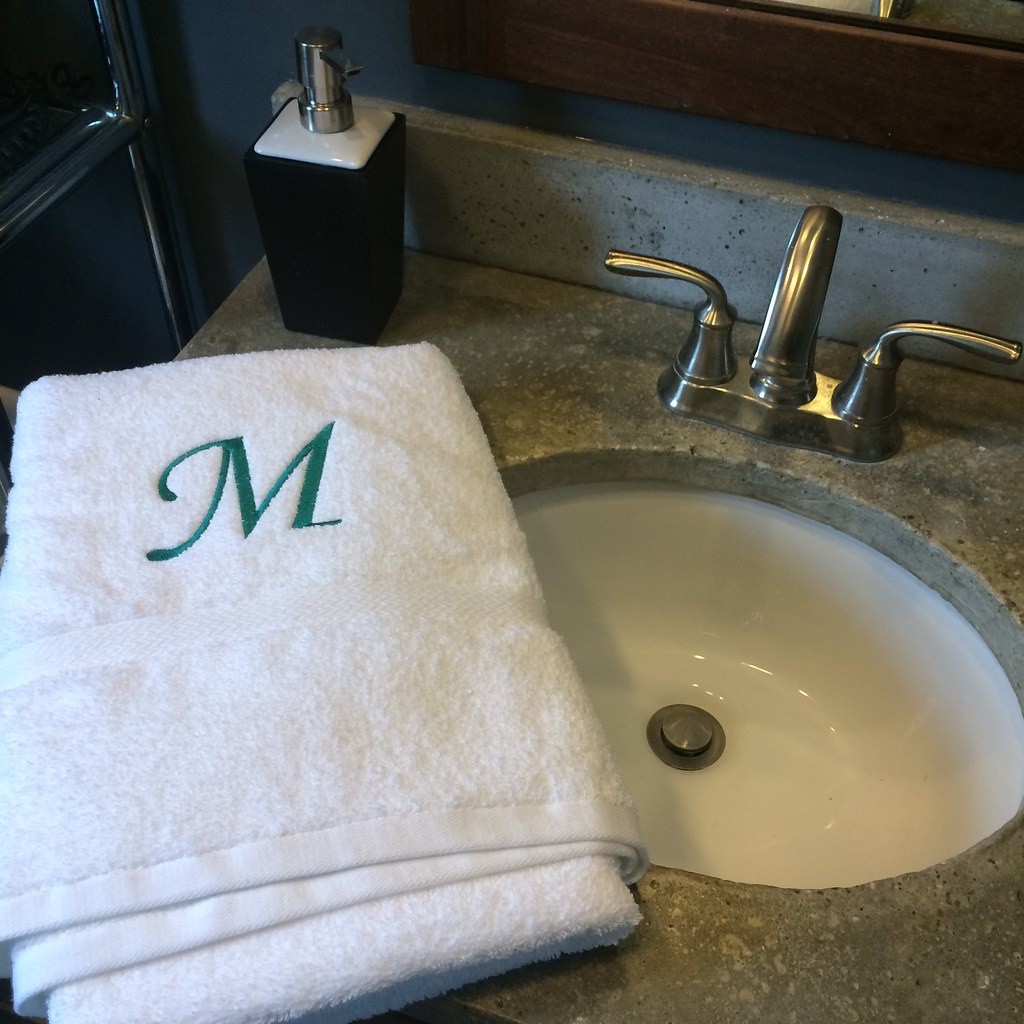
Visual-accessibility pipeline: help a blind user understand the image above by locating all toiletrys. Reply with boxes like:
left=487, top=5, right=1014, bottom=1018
left=244, top=27, right=405, bottom=343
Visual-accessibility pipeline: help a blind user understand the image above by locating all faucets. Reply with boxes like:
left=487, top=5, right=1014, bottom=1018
left=748, top=203, right=843, bottom=410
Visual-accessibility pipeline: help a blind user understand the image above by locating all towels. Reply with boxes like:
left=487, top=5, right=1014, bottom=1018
left=1, top=344, right=653, bottom=1016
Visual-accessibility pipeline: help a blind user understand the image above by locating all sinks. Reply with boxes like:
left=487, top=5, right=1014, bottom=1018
left=499, top=447, right=1024, bottom=892
